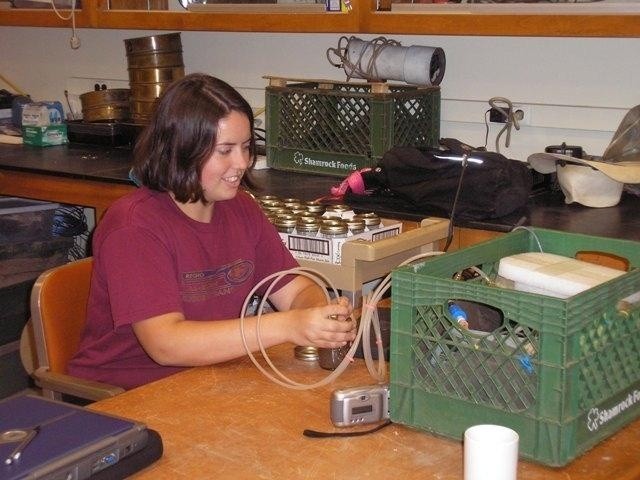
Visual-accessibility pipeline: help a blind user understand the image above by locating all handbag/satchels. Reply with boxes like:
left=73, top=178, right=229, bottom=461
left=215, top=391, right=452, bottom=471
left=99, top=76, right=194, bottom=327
left=345, top=146, right=527, bottom=223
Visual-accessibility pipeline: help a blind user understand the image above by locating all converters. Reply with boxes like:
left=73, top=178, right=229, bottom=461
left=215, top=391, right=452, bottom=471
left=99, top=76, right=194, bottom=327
left=490, top=107, right=510, bottom=123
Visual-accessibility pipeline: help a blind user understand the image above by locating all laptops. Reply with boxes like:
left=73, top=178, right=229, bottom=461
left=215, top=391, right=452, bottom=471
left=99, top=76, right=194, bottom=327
left=0, top=392, right=149, bottom=480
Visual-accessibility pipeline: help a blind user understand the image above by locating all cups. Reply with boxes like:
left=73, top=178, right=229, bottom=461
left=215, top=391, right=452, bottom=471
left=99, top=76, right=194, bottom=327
left=463, top=424, right=519, bottom=480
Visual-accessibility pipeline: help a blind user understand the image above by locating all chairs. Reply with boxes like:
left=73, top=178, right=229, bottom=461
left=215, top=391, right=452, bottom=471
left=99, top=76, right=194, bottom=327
left=17, top=257, right=131, bottom=408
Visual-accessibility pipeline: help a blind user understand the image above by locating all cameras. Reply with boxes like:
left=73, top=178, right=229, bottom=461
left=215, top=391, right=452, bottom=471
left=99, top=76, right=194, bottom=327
left=331, top=383, right=389, bottom=428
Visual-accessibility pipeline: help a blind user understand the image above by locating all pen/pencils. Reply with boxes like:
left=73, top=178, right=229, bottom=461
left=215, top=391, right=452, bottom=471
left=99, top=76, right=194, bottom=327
left=4, top=425, right=41, bottom=466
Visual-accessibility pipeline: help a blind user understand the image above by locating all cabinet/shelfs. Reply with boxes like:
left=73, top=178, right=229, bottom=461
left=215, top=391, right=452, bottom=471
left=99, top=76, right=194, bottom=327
left=359, top=0, right=640, bottom=38
left=0, top=1, right=94, bottom=31
left=94, top=0, right=359, bottom=36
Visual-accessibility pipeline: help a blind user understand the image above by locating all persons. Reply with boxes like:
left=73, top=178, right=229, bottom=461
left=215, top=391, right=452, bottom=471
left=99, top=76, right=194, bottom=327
left=62, top=73, right=357, bottom=407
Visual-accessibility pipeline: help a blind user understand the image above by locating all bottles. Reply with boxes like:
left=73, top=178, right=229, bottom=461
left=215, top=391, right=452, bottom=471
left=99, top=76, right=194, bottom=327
left=317, top=313, right=350, bottom=371
left=257, top=194, right=382, bottom=239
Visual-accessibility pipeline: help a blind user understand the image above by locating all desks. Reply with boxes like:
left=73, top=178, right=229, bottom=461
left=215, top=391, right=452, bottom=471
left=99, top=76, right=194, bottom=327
left=77, top=294, right=639, bottom=480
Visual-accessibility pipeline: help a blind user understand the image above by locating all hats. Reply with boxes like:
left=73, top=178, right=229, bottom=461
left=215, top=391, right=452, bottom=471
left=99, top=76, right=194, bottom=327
left=529, top=153, right=640, bottom=207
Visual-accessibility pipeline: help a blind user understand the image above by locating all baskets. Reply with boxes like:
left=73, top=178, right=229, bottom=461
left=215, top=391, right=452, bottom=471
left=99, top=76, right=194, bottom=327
left=264, top=81, right=440, bottom=177
left=389, top=226, right=639, bottom=469
